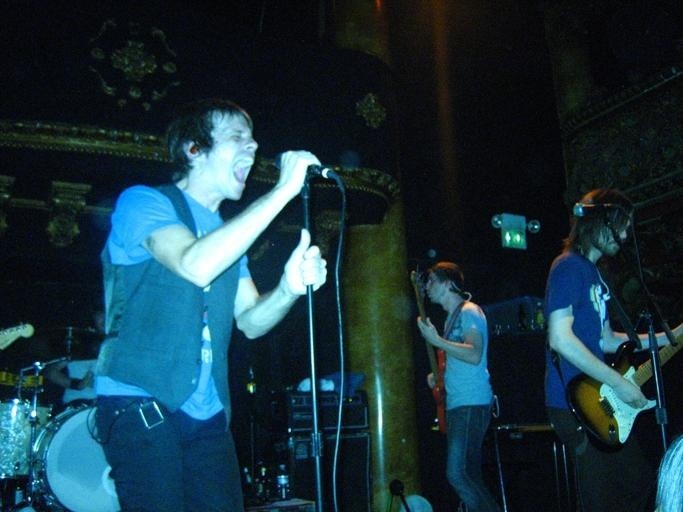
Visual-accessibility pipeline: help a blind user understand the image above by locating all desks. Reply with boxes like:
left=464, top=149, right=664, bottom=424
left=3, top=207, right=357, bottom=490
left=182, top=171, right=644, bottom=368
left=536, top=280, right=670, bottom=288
left=491, top=420, right=581, bottom=512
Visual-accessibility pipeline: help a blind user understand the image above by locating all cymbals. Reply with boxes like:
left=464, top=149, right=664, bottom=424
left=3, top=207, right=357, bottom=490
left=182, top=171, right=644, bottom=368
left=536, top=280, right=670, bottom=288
left=48, top=322, right=92, bottom=340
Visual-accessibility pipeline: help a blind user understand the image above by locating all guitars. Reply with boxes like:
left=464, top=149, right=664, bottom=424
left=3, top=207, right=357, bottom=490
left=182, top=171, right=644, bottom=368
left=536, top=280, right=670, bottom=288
left=410, top=272, right=446, bottom=433
left=565, top=333, right=682, bottom=452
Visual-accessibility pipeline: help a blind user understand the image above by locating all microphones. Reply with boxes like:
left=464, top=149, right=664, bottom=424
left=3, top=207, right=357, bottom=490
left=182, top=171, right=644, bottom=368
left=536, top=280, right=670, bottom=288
left=274, top=153, right=335, bottom=181
left=573, top=203, right=616, bottom=220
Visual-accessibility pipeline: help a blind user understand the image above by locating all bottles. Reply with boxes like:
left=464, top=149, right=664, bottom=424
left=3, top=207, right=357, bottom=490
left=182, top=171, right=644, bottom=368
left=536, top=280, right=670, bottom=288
left=13, top=463, right=26, bottom=505
left=535, top=302, right=546, bottom=330
left=241, top=465, right=289, bottom=502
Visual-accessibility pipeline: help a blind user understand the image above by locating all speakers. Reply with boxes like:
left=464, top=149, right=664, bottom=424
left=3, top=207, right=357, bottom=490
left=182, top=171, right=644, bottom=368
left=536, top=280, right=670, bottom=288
left=258, top=388, right=373, bottom=511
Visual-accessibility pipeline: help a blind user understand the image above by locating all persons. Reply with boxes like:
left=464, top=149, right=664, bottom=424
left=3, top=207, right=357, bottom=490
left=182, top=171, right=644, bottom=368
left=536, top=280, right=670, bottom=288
left=544, top=188, right=658, bottom=512
left=87, top=99, right=329, bottom=512
left=228, top=328, right=256, bottom=503
left=416, top=262, right=502, bottom=511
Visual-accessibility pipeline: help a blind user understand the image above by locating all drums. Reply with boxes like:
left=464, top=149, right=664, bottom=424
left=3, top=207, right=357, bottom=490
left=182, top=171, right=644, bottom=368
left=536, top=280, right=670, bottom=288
left=1, top=398, right=54, bottom=479
left=30, top=406, right=123, bottom=512
